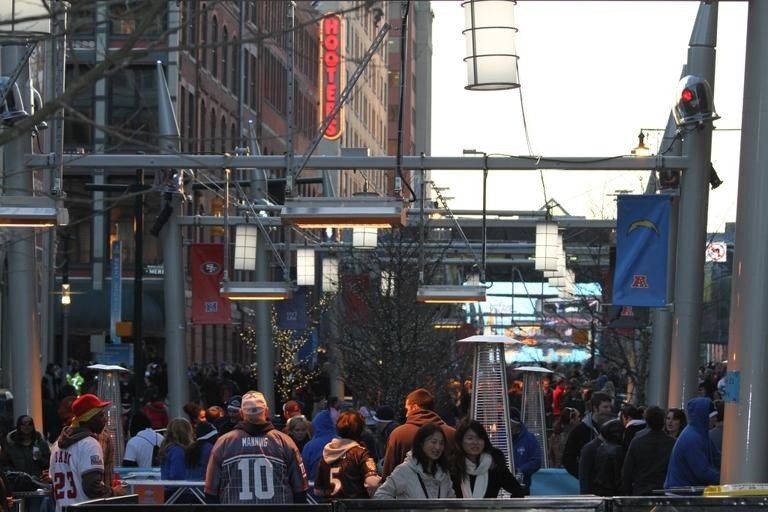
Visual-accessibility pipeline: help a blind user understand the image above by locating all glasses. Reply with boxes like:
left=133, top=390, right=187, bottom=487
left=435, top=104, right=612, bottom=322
left=20, top=421, right=33, bottom=427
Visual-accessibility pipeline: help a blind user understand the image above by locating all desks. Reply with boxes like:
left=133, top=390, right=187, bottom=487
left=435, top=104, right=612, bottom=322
left=119, top=479, right=208, bottom=505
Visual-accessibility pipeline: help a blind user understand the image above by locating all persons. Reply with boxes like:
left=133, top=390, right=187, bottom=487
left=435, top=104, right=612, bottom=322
left=0, top=358, right=127, bottom=512
left=123, top=370, right=525, bottom=512
left=507, top=368, right=729, bottom=497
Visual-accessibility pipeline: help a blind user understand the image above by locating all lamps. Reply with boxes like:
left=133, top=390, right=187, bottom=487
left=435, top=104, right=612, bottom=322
left=417, top=153, right=487, bottom=303
left=234, top=225, right=257, bottom=271
left=381, top=271, right=395, bottom=297
left=297, top=239, right=315, bottom=285
left=280, top=0, right=403, bottom=228
left=323, top=258, right=339, bottom=292
left=535, top=209, right=575, bottom=299
left=433, top=320, right=464, bottom=328
left=353, top=228, right=377, bottom=249
left=0, top=0, right=69, bottom=227
left=460, top=0, right=521, bottom=91
left=223, top=168, right=292, bottom=301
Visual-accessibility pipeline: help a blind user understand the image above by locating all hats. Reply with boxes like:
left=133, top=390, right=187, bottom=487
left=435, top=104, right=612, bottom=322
left=227, top=394, right=242, bottom=410
left=71, top=393, right=113, bottom=418
left=241, top=390, right=268, bottom=414
left=709, top=401, right=719, bottom=419
left=195, top=420, right=219, bottom=441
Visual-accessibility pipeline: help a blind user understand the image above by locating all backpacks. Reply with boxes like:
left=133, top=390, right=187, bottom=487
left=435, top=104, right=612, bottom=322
left=136, top=432, right=160, bottom=467
